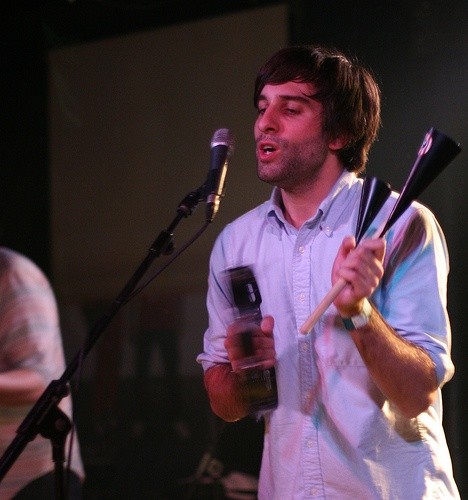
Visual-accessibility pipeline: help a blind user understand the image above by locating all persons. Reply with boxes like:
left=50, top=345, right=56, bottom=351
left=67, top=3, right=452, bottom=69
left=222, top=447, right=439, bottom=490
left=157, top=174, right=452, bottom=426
left=195, top=44, right=462, bottom=500
left=0, top=243, right=86, bottom=500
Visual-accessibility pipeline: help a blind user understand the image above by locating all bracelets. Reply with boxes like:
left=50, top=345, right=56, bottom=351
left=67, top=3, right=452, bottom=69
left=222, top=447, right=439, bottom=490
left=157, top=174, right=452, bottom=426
left=339, top=296, right=374, bottom=332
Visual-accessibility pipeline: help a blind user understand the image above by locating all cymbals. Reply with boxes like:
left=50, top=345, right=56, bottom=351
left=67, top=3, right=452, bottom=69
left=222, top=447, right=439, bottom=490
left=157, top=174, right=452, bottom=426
left=223, top=265, right=278, bottom=410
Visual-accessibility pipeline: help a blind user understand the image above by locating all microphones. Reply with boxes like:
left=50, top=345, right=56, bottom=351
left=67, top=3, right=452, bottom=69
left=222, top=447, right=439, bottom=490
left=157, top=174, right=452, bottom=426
left=228, top=267, right=277, bottom=413
left=206, top=128, right=235, bottom=221
